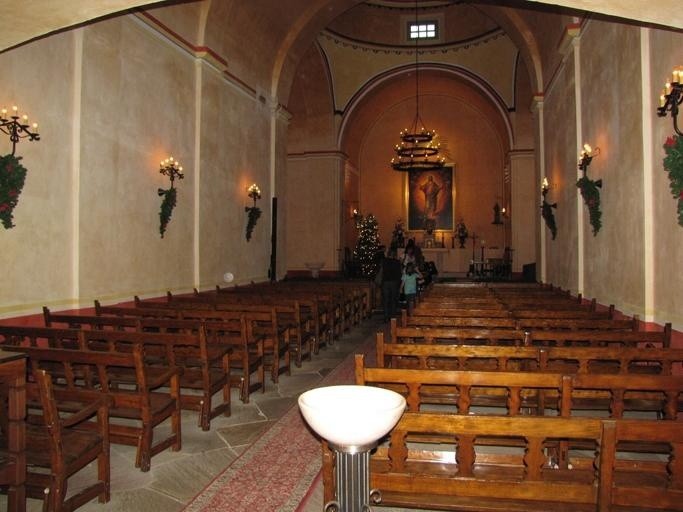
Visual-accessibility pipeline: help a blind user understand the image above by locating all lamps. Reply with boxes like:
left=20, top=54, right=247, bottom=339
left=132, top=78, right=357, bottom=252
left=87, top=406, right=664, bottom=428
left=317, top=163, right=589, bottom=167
left=574, top=142, right=603, bottom=189
left=538, top=177, right=558, bottom=209
left=0, top=106, right=41, bottom=159
left=153, top=156, right=185, bottom=196
left=244, top=183, right=263, bottom=212
left=657, top=64, right=682, bottom=136
left=389, top=1, right=445, bottom=172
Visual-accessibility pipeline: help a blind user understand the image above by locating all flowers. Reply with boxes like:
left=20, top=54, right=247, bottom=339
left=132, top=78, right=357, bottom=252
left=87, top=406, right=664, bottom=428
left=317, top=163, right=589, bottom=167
left=156, top=187, right=178, bottom=239
left=0, top=155, right=27, bottom=229
left=662, top=134, right=682, bottom=227
left=244, top=207, right=262, bottom=243
left=541, top=200, right=557, bottom=240
left=576, top=175, right=602, bottom=237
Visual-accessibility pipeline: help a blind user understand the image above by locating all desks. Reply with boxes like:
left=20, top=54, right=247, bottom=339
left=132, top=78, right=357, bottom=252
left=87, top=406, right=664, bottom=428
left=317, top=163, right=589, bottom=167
left=396, top=246, right=473, bottom=274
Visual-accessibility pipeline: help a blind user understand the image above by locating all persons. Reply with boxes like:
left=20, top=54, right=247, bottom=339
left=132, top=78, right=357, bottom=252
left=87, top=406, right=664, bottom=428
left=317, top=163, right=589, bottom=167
left=416, top=176, right=448, bottom=219
left=454, top=218, right=468, bottom=240
left=392, top=217, right=405, bottom=236
left=373, top=238, right=425, bottom=324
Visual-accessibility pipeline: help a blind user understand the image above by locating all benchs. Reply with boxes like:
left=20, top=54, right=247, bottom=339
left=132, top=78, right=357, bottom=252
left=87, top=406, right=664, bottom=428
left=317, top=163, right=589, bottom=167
left=1, top=300, right=266, bottom=512
left=322, top=278, right=682, bottom=512
left=135, top=276, right=370, bottom=384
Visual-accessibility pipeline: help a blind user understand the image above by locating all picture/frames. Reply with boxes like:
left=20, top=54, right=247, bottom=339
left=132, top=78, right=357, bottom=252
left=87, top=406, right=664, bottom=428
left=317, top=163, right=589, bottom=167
left=401, top=162, right=457, bottom=233
